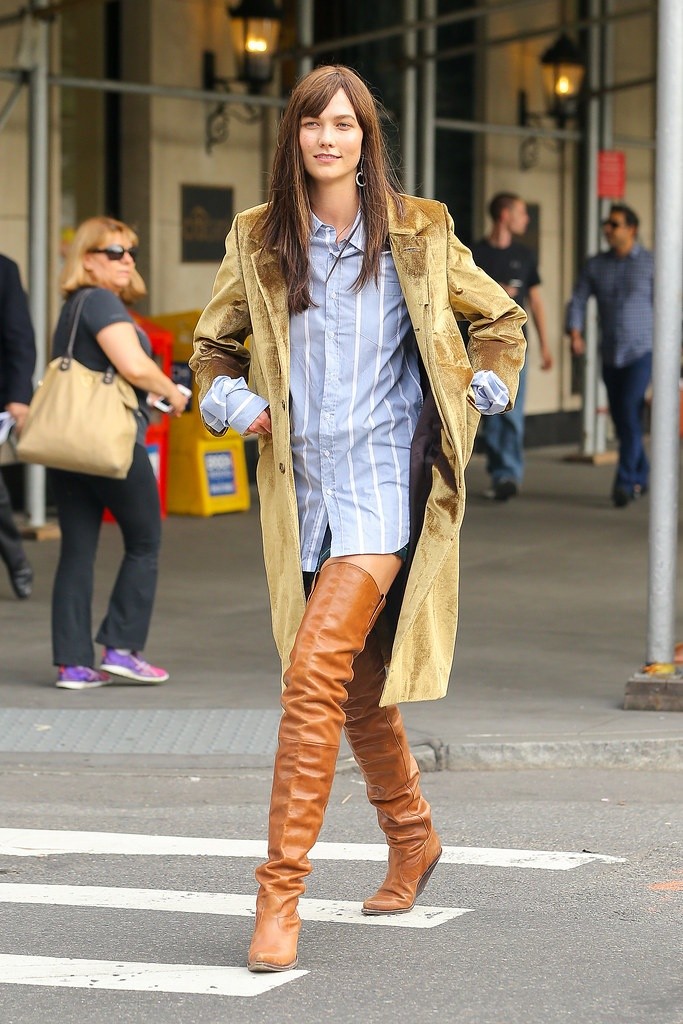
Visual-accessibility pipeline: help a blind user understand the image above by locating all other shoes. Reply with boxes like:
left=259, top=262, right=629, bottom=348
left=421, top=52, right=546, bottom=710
left=613, top=488, right=632, bottom=507
left=8, top=561, right=33, bottom=600
left=493, top=479, right=516, bottom=502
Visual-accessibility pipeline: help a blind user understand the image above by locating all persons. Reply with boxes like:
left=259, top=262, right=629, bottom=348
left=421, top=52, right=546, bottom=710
left=466, top=194, right=551, bottom=502
left=0, top=255, right=36, bottom=599
left=190, top=65, right=527, bottom=971
left=565, top=206, right=651, bottom=505
left=51, top=218, right=188, bottom=689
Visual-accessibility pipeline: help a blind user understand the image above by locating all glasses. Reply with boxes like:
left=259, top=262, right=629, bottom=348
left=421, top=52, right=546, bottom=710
left=603, top=218, right=620, bottom=229
left=87, top=244, right=142, bottom=262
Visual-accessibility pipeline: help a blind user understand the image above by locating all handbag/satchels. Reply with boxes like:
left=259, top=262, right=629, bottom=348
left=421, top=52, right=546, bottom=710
left=14, top=356, right=151, bottom=480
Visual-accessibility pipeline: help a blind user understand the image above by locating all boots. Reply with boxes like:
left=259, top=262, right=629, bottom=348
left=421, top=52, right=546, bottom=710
left=348, top=636, right=443, bottom=917
left=245, top=561, right=386, bottom=972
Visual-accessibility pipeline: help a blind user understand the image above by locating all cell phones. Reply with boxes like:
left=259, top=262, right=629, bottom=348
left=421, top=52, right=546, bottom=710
left=153, top=384, right=192, bottom=413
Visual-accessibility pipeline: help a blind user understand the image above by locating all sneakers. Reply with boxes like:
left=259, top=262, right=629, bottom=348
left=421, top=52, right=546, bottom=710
left=55, top=664, right=113, bottom=690
left=99, top=647, right=170, bottom=682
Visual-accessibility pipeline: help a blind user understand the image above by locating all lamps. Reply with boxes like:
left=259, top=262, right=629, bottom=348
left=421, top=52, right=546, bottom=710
left=517, top=30, right=590, bottom=170
left=198, top=0, right=302, bottom=156
